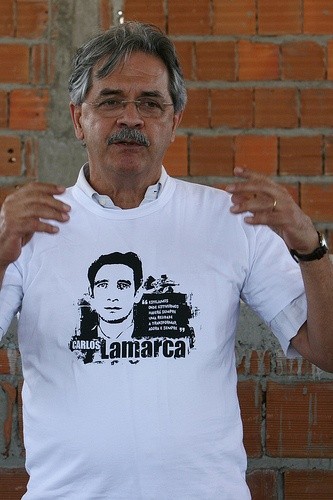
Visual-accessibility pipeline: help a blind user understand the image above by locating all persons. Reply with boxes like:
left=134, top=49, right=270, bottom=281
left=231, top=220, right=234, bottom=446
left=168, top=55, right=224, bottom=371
left=0, top=22, right=333, bottom=500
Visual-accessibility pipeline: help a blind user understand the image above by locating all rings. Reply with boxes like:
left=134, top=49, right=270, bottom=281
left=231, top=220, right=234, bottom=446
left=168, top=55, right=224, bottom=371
left=271, top=200, right=278, bottom=212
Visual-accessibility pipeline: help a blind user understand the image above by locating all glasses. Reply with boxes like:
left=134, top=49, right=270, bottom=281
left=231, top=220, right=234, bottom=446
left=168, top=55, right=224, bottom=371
left=81, top=96, right=175, bottom=119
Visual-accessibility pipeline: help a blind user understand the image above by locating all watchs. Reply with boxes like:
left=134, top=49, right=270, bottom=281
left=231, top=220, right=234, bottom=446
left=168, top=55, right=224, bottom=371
left=289, top=231, right=328, bottom=263
